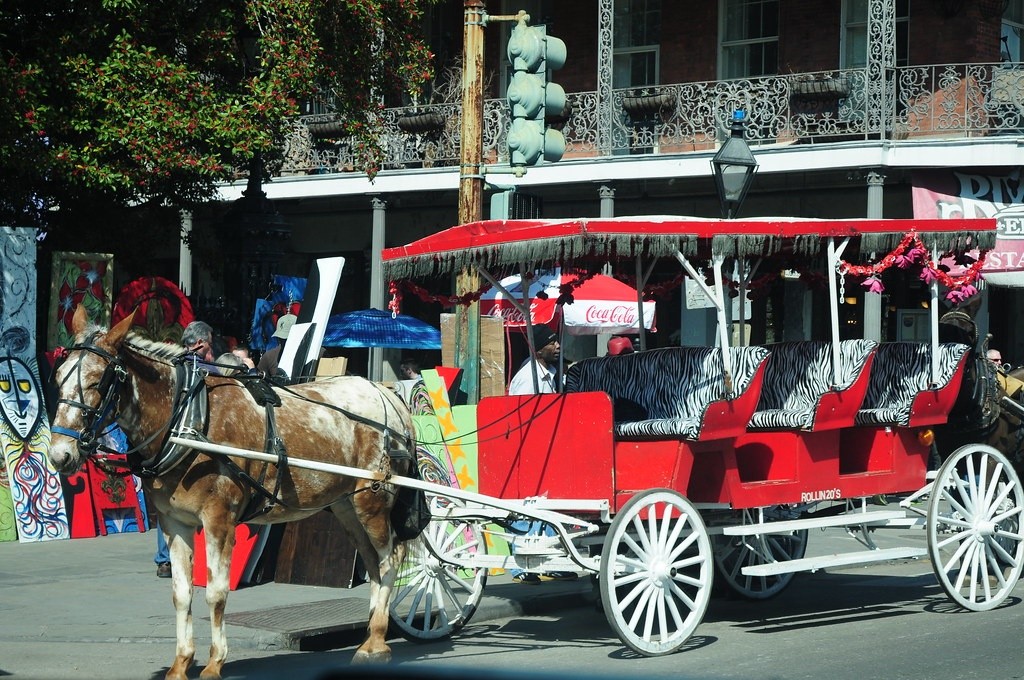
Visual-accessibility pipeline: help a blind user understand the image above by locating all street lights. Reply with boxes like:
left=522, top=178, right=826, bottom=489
left=709, top=109, right=760, bottom=350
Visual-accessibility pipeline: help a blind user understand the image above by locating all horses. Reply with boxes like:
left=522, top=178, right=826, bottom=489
left=47, top=303, right=418, bottom=680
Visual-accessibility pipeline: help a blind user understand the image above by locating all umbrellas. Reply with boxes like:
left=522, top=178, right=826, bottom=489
left=323, top=307, right=442, bottom=381
left=480, top=262, right=656, bottom=393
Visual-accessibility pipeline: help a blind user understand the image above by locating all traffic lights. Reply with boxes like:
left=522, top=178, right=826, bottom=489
left=506, top=23, right=568, bottom=167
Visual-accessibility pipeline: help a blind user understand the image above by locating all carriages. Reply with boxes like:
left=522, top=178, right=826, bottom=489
left=45, top=215, right=1024, bottom=680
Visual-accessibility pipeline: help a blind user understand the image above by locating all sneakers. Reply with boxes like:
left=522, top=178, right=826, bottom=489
left=157, top=562, right=171, bottom=577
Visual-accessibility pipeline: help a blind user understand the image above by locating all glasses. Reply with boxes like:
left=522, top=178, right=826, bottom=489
left=990, top=359, right=1002, bottom=362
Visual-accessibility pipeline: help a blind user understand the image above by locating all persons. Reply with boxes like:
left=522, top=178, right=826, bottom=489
left=400, top=358, right=423, bottom=380
left=986, top=349, right=1002, bottom=367
left=258, top=314, right=297, bottom=375
left=509, top=323, right=560, bottom=396
left=153, top=320, right=220, bottom=577
left=214, top=344, right=256, bottom=377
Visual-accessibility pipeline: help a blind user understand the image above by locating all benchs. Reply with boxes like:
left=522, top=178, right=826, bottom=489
left=562, top=340, right=972, bottom=444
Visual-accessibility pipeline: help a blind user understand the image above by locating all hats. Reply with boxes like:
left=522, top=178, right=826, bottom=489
left=271, top=314, right=297, bottom=339
left=533, top=325, right=558, bottom=352
